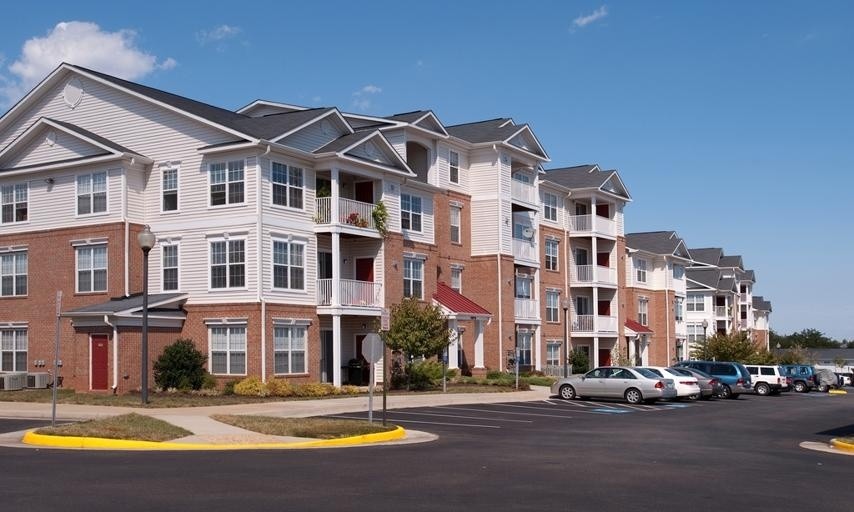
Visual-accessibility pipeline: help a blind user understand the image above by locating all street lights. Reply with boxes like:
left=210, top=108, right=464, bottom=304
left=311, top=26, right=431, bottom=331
left=701, top=319, right=708, bottom=361
left=776, top=342, right=781, bottom=365
left=136, top=224, right=156, bottom=403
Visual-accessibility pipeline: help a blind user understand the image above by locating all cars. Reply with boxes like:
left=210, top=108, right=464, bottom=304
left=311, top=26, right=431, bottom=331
left=550, top=361, right=792, bottom=404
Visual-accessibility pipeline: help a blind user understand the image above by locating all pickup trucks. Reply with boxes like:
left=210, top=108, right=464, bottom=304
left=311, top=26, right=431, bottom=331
left=781, top=365, right=844, bottom=392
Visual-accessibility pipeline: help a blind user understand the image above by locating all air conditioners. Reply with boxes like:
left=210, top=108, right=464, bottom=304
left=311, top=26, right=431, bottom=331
left=0, top=373, right=22, bottom=390
left=26, top=372, right=48, bottom=388
